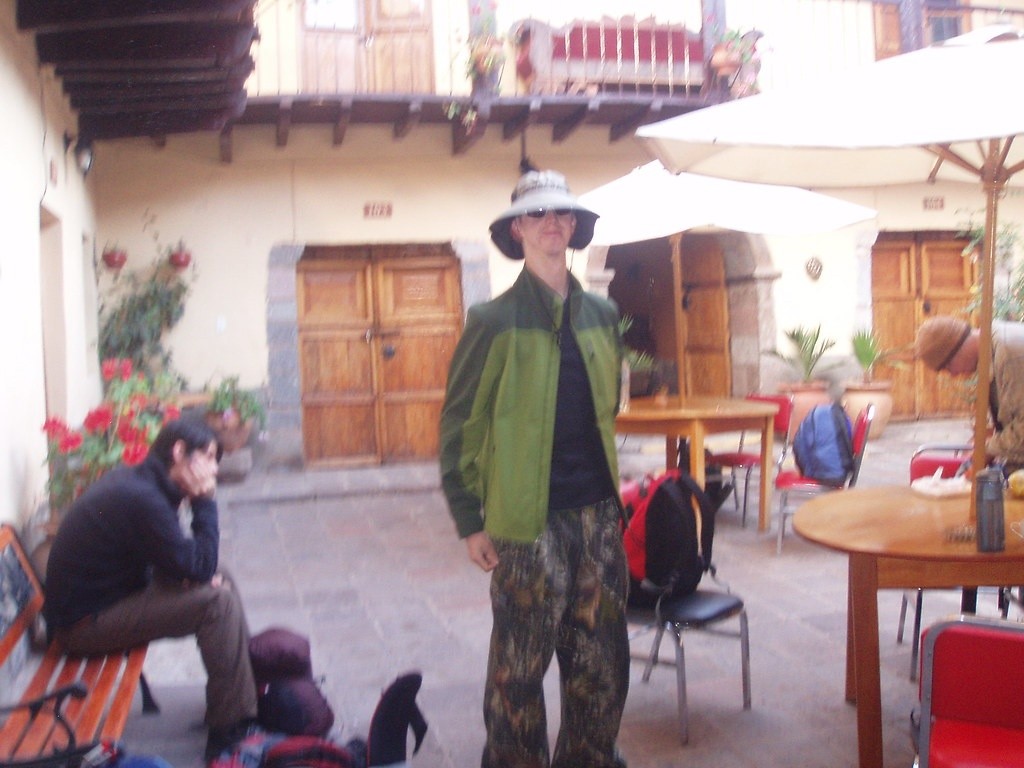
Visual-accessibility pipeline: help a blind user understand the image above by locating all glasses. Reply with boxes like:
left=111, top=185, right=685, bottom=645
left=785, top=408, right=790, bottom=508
left=525, top=205, right=573, bottom=218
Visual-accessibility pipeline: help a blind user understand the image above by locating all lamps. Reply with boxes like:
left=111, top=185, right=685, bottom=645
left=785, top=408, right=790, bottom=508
left=77, top=135, right=96, bottom=177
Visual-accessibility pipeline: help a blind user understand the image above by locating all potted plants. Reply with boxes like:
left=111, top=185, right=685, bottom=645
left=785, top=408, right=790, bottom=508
left=767, top=330, right=834, bottom=444
left=842, top=333, right=907, bottom=439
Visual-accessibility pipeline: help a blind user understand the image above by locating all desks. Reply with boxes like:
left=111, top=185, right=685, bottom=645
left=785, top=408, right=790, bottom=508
left=793, top=488, right=1024, bottom=767
left=607, top=393, right=777, bottom=535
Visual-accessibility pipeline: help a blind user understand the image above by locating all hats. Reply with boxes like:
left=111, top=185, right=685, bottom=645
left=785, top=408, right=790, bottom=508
left=489, top=169, right=599, bottom=261
left=916, top=318, right=973, bottom=373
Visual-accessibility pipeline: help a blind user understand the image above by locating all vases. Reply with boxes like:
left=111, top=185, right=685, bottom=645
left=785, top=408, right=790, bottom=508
left=29, top=515, right=63, bottom=580
left=207, top=408, right=251, bottom=452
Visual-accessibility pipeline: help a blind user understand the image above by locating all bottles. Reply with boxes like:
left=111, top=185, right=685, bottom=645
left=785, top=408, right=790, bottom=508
left=976, top=462, right=1006, bottom=553
left=619, top=357, right=631, bottom=414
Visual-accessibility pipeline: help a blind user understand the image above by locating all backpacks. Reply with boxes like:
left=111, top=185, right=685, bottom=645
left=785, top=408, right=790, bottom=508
left=791, top=401, right=857, bottom=490
left=207, top=671, right=429, bottom=766
left=248, top=628, right=334, bottom=738
left=620, top=467, right=717, bottom=611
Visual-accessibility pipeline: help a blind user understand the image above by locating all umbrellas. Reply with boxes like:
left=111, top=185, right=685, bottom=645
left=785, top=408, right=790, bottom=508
left=573, top=27, right=1024, bottom=482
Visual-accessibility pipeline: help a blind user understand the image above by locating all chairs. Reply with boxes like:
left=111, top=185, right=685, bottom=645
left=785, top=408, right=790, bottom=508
left=704, top=392, right=793, bottom=529
left=896, top=443, right=976, bottom=640
left=910, top=613, right=1024, bottom=768
left=774, top=405, right=877, bottom=548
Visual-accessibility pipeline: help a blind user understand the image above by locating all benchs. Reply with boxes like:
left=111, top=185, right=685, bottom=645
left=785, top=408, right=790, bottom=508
left=507, top=17, right=716, bottom=92
left=0, top=524, right=161, bottom=768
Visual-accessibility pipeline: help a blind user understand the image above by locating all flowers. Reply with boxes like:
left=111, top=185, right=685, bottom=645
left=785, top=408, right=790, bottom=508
left=34, top=250, right=194, bottom=524
left=208, top=376, right=268, bottom=442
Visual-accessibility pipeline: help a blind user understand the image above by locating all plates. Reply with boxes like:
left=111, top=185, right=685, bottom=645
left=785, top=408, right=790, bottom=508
left=911, top=477, right=972, bottom=497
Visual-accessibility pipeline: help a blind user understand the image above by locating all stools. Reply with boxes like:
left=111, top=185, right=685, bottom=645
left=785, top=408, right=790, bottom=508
left=624, top=589, right=750, bottom=751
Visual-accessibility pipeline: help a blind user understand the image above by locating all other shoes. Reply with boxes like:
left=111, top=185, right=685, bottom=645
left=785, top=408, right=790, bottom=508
left=204, top=720, right=257, bottom=761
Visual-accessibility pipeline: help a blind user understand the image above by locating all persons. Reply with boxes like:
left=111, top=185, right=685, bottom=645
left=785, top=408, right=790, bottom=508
left=438, top=171, right=632, bottom=768
left=41, top=419, right=260, bottom=761
left=915, top=315, right=1024, bottom=483
